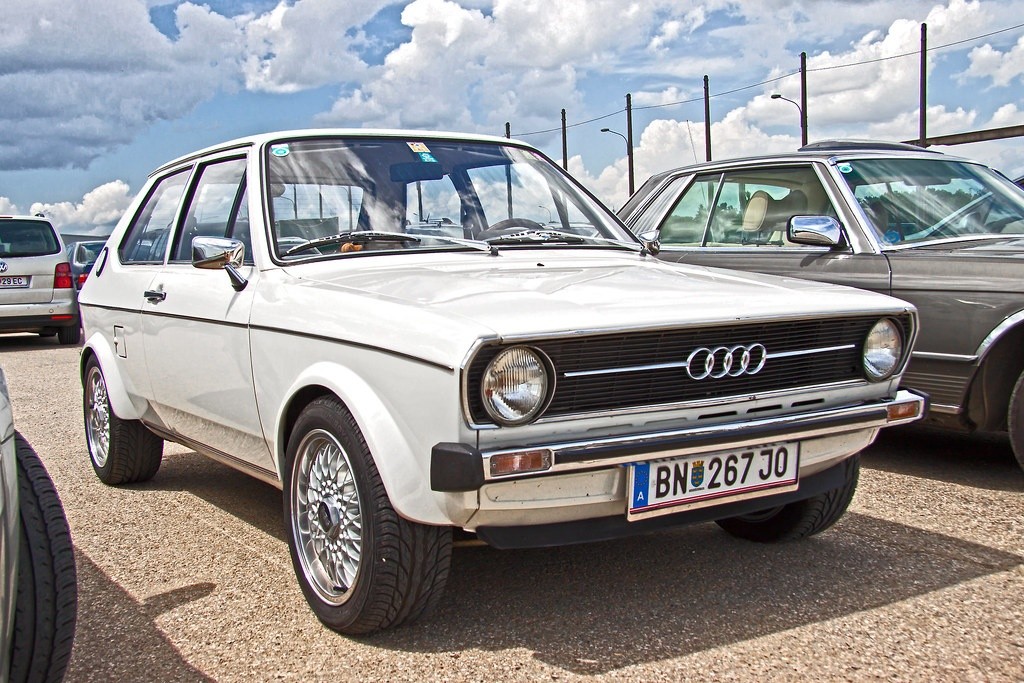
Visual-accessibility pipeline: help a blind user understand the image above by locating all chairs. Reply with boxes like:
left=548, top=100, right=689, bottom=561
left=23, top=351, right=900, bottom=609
left=1, top=214, right=1024, bottom=256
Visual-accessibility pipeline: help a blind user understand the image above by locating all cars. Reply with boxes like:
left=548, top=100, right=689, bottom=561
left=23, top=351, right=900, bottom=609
left=591, top=138, right=1024, bottom=479
left=71, top=128, right=931, bottom=640
left=65, top=239, right=107, bottom=291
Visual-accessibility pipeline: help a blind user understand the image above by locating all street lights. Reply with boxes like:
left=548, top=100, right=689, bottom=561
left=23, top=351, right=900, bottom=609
left=770, top=94, right=810, bottom=147
left=601, top=127, right=639, bottom=196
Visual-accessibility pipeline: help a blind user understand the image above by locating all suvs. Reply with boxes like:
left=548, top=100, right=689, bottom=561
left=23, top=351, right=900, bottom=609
left=0, top=212, right=80, bottom=345
